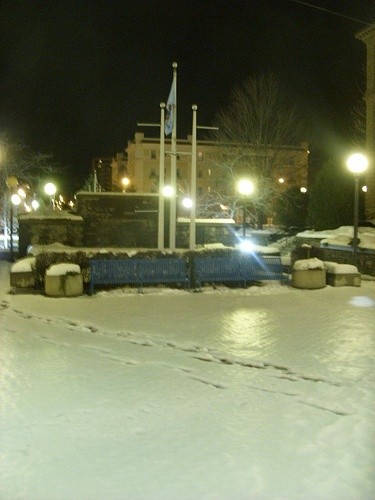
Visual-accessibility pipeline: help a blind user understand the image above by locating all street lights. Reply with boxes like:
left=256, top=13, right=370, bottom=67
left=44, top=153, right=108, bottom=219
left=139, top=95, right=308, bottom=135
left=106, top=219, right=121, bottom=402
left=346, top=152, right=370, bottom=267
left=44, top=183, right=56, bottom=210
left=237, top=180, right=254, bottom=239
left=6, top=176, right=18, bottom=262
